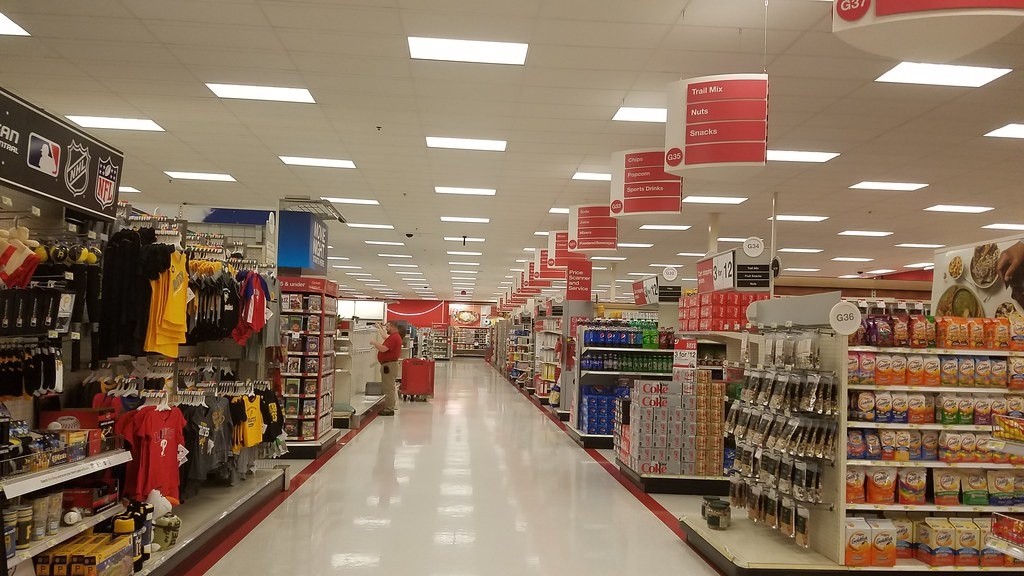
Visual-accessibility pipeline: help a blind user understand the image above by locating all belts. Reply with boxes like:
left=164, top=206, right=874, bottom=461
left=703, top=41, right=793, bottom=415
left=381, top=360, right=397, bottom=365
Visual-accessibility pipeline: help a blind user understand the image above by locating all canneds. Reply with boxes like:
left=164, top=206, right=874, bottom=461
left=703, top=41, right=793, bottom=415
left=714, top=500, right=731, bottom=526
left=702, top=496, right=720, bottom=520
left=707, top=504, right=728, bottom=530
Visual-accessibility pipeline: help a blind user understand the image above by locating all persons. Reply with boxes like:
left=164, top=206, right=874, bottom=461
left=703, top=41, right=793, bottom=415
left=995, top=238, right=1024, bottom=281
left=370, top=320, right=406, bottom=416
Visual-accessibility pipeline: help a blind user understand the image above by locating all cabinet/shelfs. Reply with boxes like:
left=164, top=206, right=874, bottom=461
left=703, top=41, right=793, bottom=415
left=0, top=447, right=132, bottom=569
left=276, top=277, right=325, bottom=440
left=334, top=322, right=489, bottom=415
left=317, top=280, right=337, bottom=438
left=492, top=319, right=1024, bottom=572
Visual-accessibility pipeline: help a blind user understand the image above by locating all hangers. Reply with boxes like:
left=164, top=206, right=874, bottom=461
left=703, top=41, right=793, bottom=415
left=83, top=357, right=271, bottom=412
left=116, top=203, right=278, bottom=278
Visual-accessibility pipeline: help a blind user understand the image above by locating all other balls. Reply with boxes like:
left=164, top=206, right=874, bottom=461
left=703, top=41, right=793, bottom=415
left=31, top=244, right=103, bottom=265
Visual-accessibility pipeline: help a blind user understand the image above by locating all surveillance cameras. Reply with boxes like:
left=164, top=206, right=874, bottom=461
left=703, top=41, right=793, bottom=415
left=857, top=271, right=864, bottom=275
left=424, top=287, right=427, bottom=288
left=406, top=234, right=413, bottom=237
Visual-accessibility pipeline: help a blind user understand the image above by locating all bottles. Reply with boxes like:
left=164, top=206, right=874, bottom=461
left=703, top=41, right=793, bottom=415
left=619, top=354, right=672, bottom=373
left=661, top=325, right=674, bottom=348
left=583, top=353, right=617, bottom=372
left=578, top=317, right=659, bottom=349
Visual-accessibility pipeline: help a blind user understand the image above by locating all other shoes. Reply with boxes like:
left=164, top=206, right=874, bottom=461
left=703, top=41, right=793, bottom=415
left=378, top=410, right=394, bottom=416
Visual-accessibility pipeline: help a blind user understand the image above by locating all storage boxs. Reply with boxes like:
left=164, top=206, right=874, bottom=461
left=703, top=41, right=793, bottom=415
left=678, top=292, right=769, bottom=335
left=34, top=531, right=134, bottom=576
